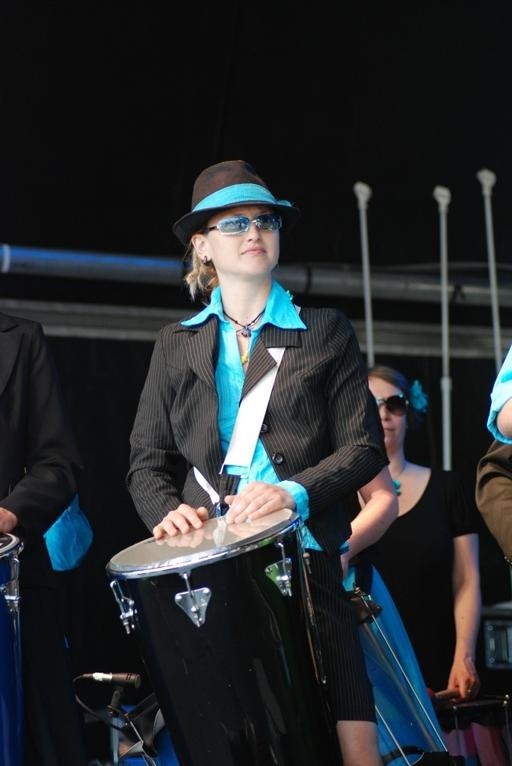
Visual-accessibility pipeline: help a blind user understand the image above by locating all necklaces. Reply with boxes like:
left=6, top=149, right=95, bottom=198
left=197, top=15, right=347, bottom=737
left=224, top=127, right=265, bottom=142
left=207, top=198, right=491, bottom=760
left=392, top=458, right=407, bottom=496
left=219, top=307, right=268, bottom=338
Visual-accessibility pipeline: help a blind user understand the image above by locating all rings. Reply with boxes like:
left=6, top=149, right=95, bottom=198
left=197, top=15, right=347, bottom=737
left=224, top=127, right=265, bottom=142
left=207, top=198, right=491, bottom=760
left=467, top=688, right=471, bottom=694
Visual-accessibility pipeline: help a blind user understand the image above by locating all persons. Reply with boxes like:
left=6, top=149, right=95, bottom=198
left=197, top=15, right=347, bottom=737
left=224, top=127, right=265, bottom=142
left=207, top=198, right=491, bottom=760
left=484, top=343, right=512, bottom=448
left=335, top=466, right=448, bottom=766
left=361, top=360, right=483, bottom=709
left=126, top=158, right=387, bottom=766
left=471, top=434, right=510, bottom=561
left=0, top=314, right=86, bottom=766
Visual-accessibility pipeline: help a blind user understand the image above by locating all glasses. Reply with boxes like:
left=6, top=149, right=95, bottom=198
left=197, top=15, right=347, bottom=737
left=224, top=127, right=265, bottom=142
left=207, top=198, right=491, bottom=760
left=376, top=393, right=410, bottom=417
left=199, top=213, right=283, bottom=236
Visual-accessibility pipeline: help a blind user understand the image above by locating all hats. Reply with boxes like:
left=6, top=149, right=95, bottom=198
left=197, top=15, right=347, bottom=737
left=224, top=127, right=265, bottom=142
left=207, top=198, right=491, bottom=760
left=170, top=159, right=302, bottom=249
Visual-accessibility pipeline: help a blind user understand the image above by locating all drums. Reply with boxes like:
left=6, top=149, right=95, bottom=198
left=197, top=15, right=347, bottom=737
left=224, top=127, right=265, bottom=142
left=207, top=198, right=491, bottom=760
left=0, top=533, right=27, bottom=764
left=106, top=508, right=341, bottom=765
